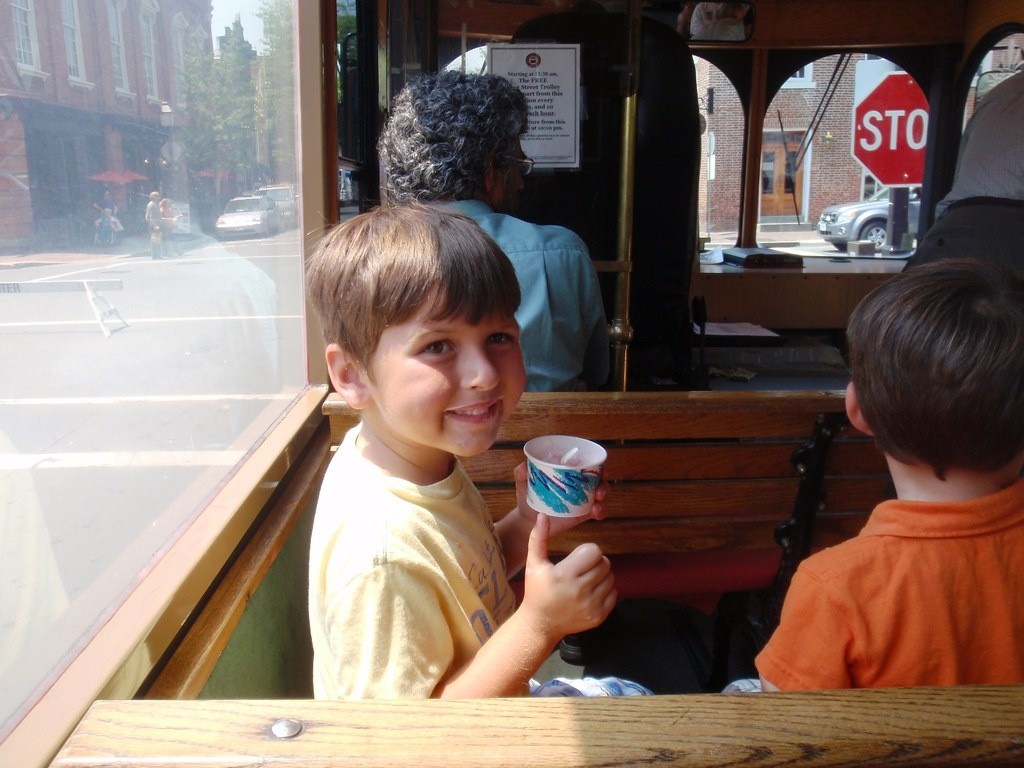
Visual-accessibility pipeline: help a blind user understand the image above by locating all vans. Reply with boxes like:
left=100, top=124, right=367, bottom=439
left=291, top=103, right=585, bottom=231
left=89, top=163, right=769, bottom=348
left=253, top=184, right=296, bottom=222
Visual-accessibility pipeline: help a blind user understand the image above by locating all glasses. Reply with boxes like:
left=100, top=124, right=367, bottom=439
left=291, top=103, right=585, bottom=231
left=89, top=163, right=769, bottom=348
left=505, top=155, right=535, bottom=176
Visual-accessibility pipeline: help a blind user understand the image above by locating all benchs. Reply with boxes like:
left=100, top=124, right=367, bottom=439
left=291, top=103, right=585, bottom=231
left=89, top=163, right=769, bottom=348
left=47, top=685, right=1024, bottom=768
left=319, top=392, right=898, bottom=691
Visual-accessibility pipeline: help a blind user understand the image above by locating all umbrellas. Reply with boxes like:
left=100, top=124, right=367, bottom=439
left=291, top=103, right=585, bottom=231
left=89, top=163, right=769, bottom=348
left=86, top=167, right=150, bottom=218
left=190, top=165, right=227, bottom=179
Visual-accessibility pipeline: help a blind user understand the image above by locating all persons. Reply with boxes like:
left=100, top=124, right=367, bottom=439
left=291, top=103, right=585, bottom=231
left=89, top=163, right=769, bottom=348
left=373, top=70, right=613, bottom=396
left=894, top=71, right=1024, bottom=271
left=302, top=201, right=660, bottom=701
left=726, top=258, right=1024, bottom=695
left=145, top=192, right=184, bottom=260
left=93, top=190, right=123, bottom=248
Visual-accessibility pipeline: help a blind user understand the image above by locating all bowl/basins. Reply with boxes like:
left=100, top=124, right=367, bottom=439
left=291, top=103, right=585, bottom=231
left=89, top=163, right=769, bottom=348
left=524, top=434, right=608, bottom=519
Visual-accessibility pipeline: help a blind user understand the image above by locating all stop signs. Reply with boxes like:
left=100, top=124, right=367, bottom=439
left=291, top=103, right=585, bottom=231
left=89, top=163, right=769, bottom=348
left=851, top=72, right=930, bottom=189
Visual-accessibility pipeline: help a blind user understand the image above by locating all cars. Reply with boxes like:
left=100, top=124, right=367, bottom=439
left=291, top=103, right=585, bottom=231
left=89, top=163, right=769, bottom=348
left=820, top=185, right=922, bottom=253
left=214, top=194, right=281, bottom=240
